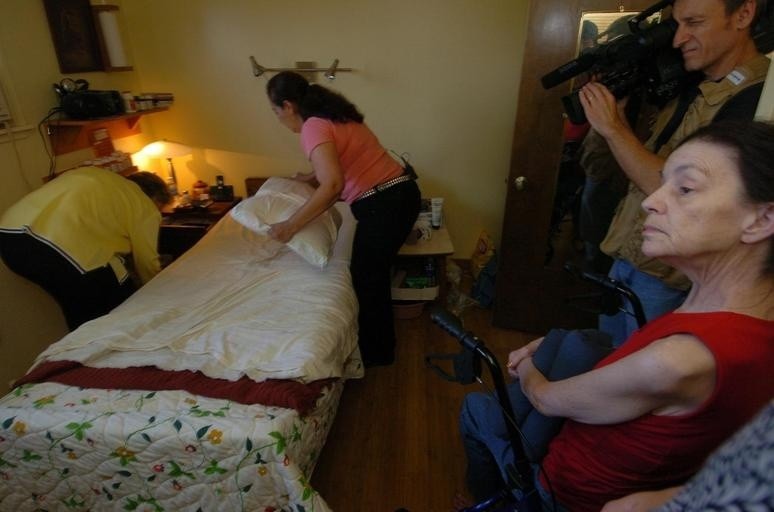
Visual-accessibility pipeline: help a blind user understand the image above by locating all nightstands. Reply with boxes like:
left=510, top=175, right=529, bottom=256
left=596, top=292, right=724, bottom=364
left=160, top=195, right=243, bottom=253
left=397, top=219, right=455, bottom=309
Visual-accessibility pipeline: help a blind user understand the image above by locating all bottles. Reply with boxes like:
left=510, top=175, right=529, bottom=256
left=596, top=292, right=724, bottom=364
left=425, top=263, right=437, bottom=288
left=122, top=91, right=154, bottom=115
left=179, top=180, right=209, bottom=205
left=85, top=151, right=132, bottom=175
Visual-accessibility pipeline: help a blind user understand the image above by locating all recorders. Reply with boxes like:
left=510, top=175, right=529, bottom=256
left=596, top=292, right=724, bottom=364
left=61, top=90, right=125, bottom=118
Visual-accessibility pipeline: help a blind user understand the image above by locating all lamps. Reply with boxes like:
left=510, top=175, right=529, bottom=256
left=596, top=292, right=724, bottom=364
left=248, top=55, right=353, bottom=81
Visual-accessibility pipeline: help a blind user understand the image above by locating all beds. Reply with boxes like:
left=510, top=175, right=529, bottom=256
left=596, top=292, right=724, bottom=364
left=0, top=176, right=364, bottom=512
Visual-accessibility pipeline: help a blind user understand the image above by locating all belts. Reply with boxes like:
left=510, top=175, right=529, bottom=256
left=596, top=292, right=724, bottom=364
left=353, top=172, right=412, bottom=201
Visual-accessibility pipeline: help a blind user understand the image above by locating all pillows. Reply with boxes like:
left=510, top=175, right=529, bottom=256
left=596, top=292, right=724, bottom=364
left=231, top=176, right=343, bottom=267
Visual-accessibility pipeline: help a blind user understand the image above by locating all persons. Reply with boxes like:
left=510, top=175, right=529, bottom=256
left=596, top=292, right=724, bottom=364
left=0, top=167, right=171, bottom=330
left=267, top=68, right=421, bottom=368
left=461, top=0, right=774, bottom=511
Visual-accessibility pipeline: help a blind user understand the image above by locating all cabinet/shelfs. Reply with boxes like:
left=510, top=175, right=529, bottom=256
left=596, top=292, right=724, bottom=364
left=492, top=0, right=673, bottom=334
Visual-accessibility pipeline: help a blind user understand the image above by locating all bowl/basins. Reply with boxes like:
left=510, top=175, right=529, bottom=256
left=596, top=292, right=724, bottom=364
left=392, top=300, right=426, bottom=320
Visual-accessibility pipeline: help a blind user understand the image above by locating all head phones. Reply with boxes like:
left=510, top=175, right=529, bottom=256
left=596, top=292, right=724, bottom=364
left=52, top=77, right=91, bottom=96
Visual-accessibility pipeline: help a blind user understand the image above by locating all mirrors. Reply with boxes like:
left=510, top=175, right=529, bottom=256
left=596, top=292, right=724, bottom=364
left=542, top=11, right=661, bottom=267
